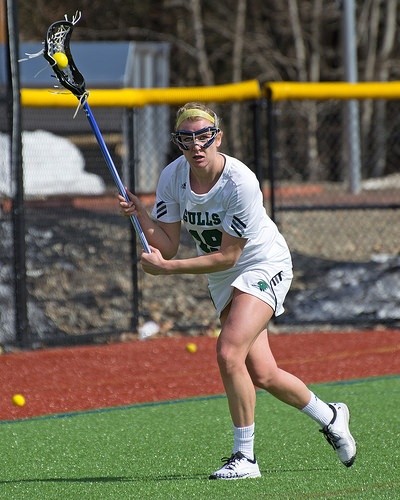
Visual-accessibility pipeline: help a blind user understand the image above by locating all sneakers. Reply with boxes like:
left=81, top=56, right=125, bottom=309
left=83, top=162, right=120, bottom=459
left=319, top=402, right=357, bottom=467
left=208, top=450, right=262, bottom=480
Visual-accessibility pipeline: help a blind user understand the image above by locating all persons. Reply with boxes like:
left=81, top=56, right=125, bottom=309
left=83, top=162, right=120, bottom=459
left=118, top=102, right=358, bottom=480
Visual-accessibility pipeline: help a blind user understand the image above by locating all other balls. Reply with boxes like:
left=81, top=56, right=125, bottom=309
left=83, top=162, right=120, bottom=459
left=14, top=392, right=25, bottom=407
left=187, top=343, right=198, bottom=353
left=53, top=53, right=68, bottom=70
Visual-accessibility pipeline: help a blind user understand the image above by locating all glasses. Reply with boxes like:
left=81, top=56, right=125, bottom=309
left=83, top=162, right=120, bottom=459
left=169, top=126, right=220, bottom=150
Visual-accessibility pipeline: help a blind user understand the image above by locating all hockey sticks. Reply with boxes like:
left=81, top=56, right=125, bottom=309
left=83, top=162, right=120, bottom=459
left=43, top=12, right=153, bottom=256
